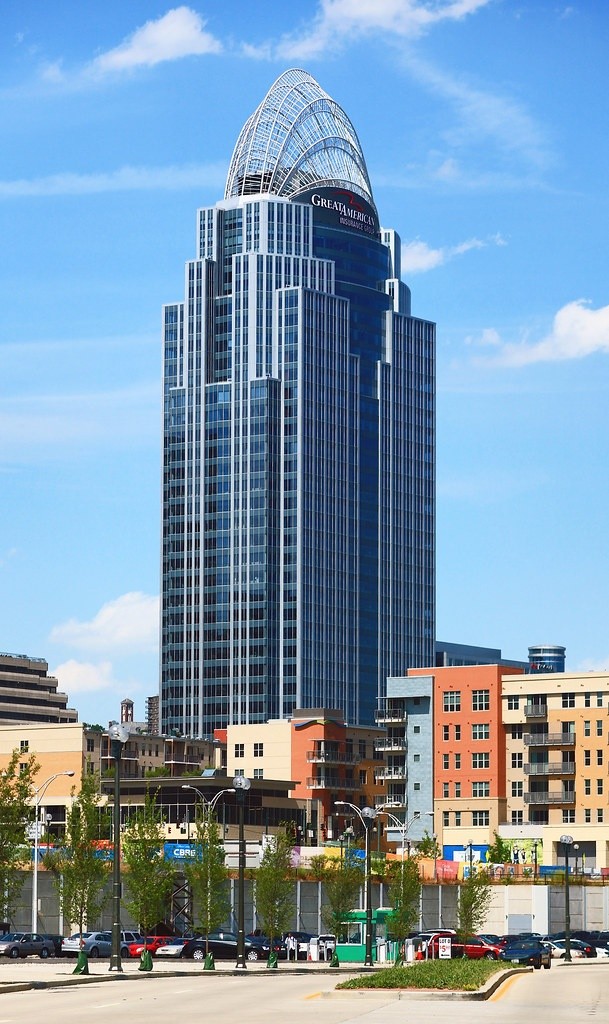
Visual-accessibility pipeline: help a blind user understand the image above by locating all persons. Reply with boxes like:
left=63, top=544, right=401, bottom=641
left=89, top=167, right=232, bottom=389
left=284, top=933, right=296, bottom=960
left=512, top=845, right=536, bottom=864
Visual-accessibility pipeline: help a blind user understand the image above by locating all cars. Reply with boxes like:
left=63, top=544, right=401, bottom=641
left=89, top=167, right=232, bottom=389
left=1, top=928, right=609, bottom=961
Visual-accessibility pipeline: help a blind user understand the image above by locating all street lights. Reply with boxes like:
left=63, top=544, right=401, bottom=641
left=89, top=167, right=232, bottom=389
left=179, top=784, right=236, bottom=955
left=45, top=814, right=52, bottom=859
left=334, top=800, right=401, bottom=945
left=376, top=811, right=435, bottom=907
left=574, top=844, right=579, bottom=875
left=107, top=723, right=129, bottom=973
left=560, top=835, right=574, bottom=962
left=532, top=839, right=539, bottom=882
left=361, top=807, right=376, bottom=967
left=468, top=839, right=473, bottom=878
left=0, top=771, right=76, bottom=940
left=232, top=775, right=251, bottom=969
